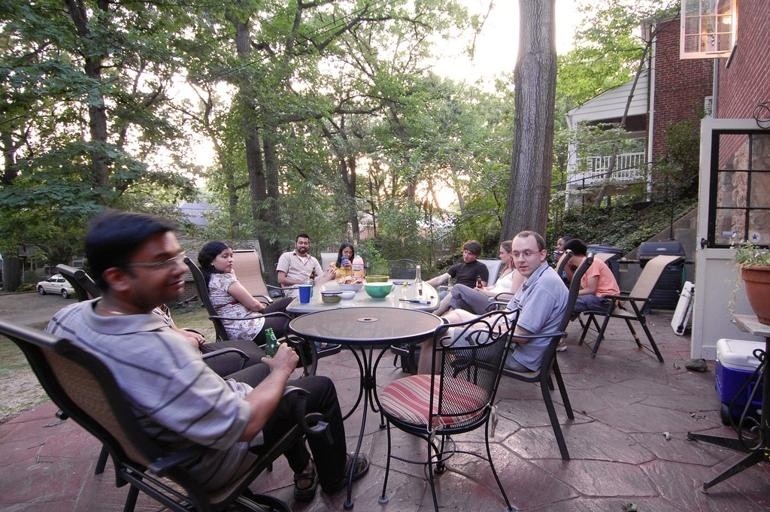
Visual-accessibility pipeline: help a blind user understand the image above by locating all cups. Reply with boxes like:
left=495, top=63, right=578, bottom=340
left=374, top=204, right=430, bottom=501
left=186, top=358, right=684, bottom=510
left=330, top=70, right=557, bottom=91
left=298, top=285, right=313, bottom=305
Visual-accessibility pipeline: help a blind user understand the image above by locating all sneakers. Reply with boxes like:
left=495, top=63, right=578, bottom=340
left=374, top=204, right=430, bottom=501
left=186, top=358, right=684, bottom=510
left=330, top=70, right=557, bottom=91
left=556, top=337, right=567, bottom=352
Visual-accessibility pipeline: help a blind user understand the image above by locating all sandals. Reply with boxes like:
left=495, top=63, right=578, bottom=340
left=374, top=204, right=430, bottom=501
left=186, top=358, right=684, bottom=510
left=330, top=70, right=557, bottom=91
left=293, top=458, right=319, bottom=502
left=323, top=452, right=370, bottom=491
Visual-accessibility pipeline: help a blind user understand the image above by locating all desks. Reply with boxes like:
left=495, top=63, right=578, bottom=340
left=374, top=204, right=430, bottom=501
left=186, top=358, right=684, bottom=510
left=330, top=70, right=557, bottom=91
left=288, top=307, right=445, bottom=512
left=685, top=314, right=770, bottom=492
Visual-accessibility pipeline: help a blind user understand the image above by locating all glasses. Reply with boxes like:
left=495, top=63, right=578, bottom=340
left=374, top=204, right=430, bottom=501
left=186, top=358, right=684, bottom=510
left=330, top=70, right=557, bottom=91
left=127, top=250, right=187, bottom=270
left=511, top=250, right=541, bottom=258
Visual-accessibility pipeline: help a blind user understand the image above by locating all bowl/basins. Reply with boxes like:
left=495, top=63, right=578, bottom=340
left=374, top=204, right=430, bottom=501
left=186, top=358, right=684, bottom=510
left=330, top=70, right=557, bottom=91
left=339, top=283, right=363, bottom=293
left=338, top=290, right=356, bottom=300
left=365, top=274, right=389, bottom=283
left=364, top=282, right=394, bottom=299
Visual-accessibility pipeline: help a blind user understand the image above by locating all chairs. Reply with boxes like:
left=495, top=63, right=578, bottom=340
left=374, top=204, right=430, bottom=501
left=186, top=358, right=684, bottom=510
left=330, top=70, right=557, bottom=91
left=55, top=262, right=104, bottom=303
left=379, top=308, right=521, bottom=512
left=322, top=250, right=366, bottom=273
left=579, top=254, right=683, bottom=362
left=448, top=250, right=593, bottom=461
left=184, top=257, right=344, bottom=372
left=226, top=246, right=289, bottom=303
left=484, top=248, right=573, bottom=316
left=0, top=322, right=334, bottom=512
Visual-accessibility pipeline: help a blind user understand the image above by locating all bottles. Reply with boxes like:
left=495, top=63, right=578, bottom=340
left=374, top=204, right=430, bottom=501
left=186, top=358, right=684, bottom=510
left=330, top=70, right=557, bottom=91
left=310, top=267, right=317, bottom=286
left=477, top=276, right=482, bottom=288
left=265, top=328, right=281, bottom=359
left=413, top=266, right=423, bottom=299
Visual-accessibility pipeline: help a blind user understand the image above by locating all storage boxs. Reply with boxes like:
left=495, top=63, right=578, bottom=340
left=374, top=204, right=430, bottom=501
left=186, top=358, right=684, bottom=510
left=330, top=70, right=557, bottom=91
left=713, top=338, right=770, bottom=409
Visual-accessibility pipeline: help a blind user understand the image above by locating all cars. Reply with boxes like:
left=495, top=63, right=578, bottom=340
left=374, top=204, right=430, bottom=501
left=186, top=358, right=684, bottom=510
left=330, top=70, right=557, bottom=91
left=35, top=274, right=76, bottom=298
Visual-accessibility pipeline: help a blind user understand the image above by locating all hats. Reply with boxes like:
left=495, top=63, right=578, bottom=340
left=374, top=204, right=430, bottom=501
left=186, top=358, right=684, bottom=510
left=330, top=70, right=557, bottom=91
left=464, top=240, right=481, bottom=257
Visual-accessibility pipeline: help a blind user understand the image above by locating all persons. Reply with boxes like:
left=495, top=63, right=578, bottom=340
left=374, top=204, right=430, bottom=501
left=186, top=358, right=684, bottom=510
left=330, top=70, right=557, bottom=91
left=432, top=240, right=525, bottom=316
left=42, top=211, right=371, bottom=504
left=555, top=240, right=621, bottom=352
left=415, top=230, right=569, bottom=375
left=327, top=243, right=364, bottom=281
left=555, top=236, right=575, bottom=262
left=277, top=233, right=324, bottom=299
left=197, top=241, right=341, bottom=368
left=426, top=239, right=489, bottom=302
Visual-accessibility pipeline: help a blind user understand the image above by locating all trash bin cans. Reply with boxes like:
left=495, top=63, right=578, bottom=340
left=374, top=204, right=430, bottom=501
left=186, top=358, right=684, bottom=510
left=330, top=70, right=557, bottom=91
left=639, top=241, right=686, bottom=310
left=587, top=244, right=624, bottom=286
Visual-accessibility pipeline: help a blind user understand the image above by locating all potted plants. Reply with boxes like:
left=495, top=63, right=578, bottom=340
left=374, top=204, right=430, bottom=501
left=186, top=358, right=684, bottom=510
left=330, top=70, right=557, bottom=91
left=739, top=244, right=770, bottom=324
